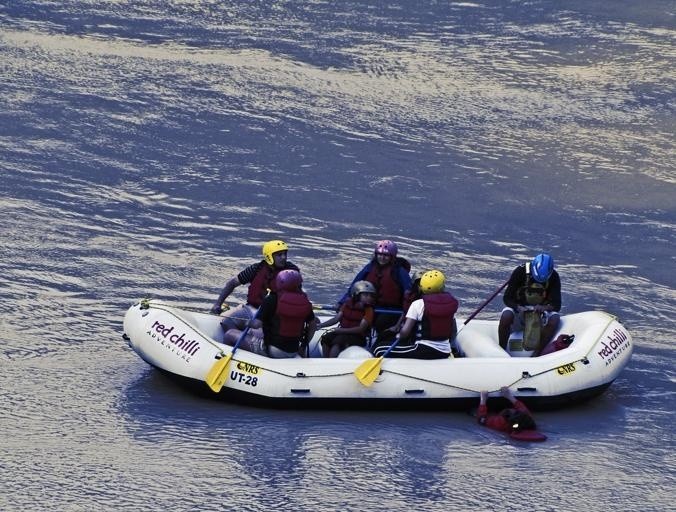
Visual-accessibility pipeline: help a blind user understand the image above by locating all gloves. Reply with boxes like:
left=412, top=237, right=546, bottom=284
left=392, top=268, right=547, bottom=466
left=123, top=298, right=632, bottom=411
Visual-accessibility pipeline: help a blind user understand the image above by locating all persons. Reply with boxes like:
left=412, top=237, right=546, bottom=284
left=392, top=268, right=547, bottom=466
left=211, top=239, right=465, bottom=358
left=477, top=385, right=538, bottom=433
left=497, top=253, right=562, bottom=358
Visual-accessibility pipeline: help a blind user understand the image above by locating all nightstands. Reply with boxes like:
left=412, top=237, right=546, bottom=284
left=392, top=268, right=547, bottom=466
left=456, top=279, right=512, bottom=335
left=354, top=339, right=399, bottom=386
left=206, top=288, right=271, bottom=393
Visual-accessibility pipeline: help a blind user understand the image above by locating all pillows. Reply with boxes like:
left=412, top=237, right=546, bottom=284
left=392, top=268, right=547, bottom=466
left=351, top=280, right=376, bottom=300
left=276, top=270, right=302, bottom=291
left=531, top=253, right=554, bottom=282
left=412, top=270, right=445, bottom=294
left=263, top=239, right=288, bottom=266
left=376, top=240, right=397, bottom=255
